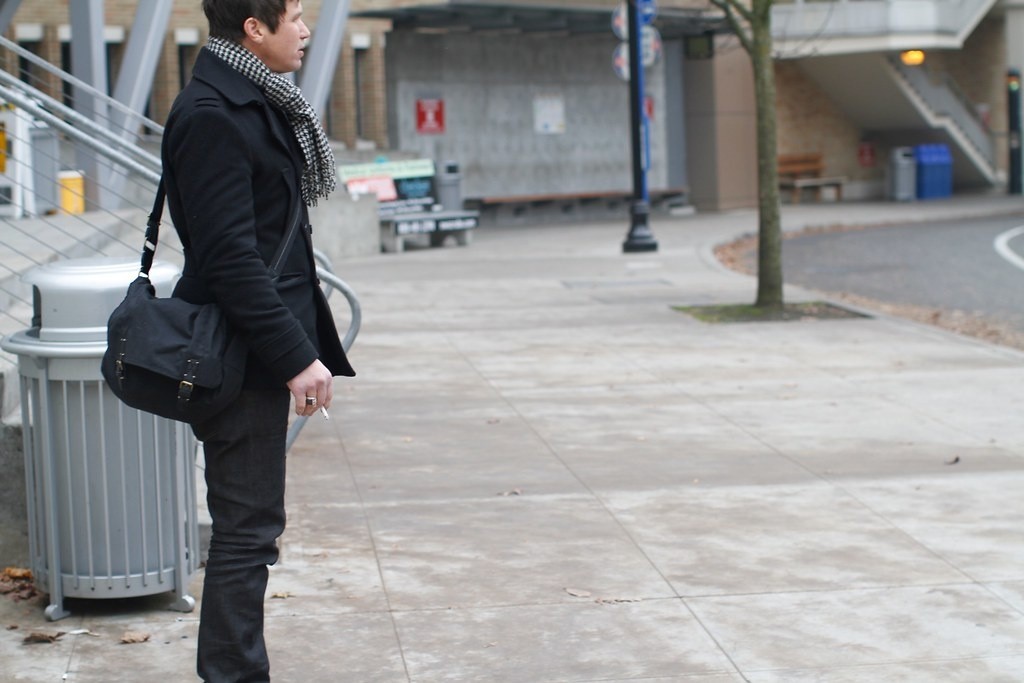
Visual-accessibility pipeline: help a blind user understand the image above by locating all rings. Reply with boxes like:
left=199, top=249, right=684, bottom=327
left=306, top=397, right=316, bottom=406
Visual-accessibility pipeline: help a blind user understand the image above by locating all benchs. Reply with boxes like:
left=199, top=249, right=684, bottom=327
left=380, top=210, right=481, bottom=255
left=779, top=152, right=843, bottom=204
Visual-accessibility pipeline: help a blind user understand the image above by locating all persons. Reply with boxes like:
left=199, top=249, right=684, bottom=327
left=161, top=0, right=356, bottom=683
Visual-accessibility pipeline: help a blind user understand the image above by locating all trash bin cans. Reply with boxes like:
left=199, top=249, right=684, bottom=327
left=433, top=158, right=465, bottom=211
left=882, top=144, right=918, bottom=202
left=917, top=144, right=953, bottom=198
left=0, top=262, right=198, bottom=621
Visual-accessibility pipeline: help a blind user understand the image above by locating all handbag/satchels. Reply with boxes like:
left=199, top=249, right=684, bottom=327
left=101, top=276, right=248, bottom=424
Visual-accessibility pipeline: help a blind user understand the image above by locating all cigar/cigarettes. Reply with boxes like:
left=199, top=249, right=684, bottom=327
left=320, top=406, right=329, bottom=420
